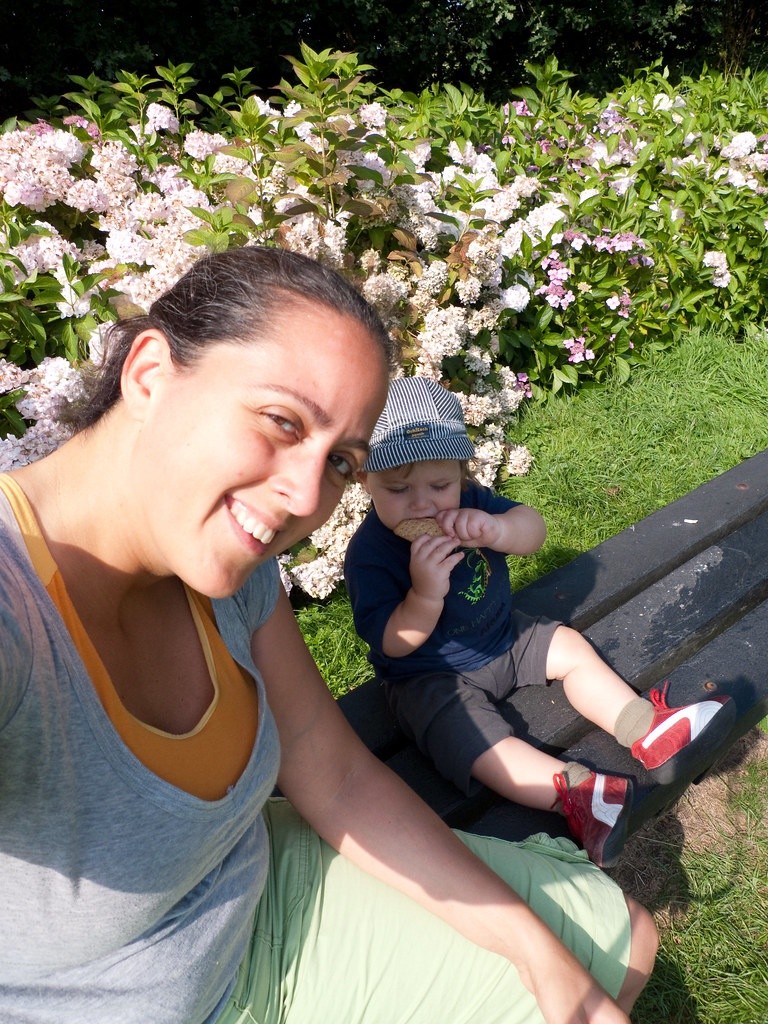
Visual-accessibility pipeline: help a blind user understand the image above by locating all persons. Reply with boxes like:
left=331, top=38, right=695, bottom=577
left=342, top=375, right=739, bottom=867
left=0, top=245, right=660, bottom=1024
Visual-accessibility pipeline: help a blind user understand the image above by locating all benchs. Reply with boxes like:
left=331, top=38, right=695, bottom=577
left=338, top=445, right=768, bottom=841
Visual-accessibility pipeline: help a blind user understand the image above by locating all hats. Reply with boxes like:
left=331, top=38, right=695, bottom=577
left=364, top=376, right=474, bottom=472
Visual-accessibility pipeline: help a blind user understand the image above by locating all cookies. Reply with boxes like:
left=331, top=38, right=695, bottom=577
left=394, top=518, right=446, bottom=544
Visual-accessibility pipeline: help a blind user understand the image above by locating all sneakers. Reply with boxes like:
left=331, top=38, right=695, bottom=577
left=631, top=680, right=737, bottom=786
left=549, top=770, right=633, bottom=866
